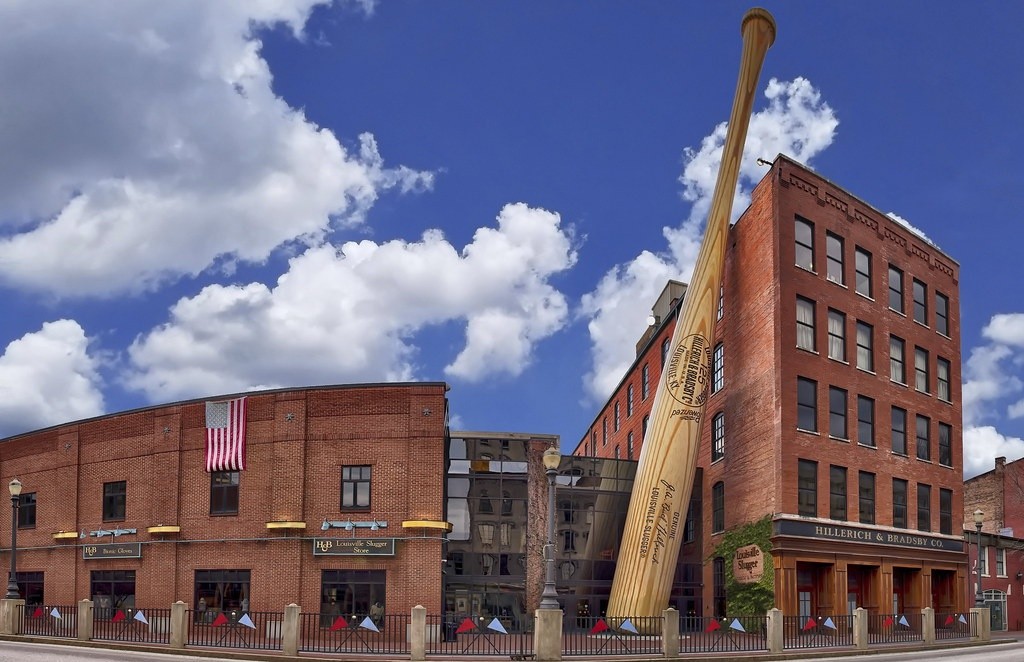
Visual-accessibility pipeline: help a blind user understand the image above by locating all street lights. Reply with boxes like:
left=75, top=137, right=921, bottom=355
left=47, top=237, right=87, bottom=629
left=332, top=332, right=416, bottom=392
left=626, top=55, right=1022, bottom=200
left=540, top=442, right=561, bottom=609
left=5, top=477, right=22, bottom=599
left=972, top=507, right=987, bottom=609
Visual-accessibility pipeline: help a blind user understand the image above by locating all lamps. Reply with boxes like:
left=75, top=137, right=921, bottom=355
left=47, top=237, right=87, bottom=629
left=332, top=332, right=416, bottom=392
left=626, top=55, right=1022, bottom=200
left=371, top=518, right=379, bottom=530
left=321, top=518, right=333, bottom=530
left=345, top=517, right=356, bottom=530
left=97, top=526, right=114, bottom=538
left=79, top=528, right=97, bottom=538
left=115, top=525, right=131, bottom=536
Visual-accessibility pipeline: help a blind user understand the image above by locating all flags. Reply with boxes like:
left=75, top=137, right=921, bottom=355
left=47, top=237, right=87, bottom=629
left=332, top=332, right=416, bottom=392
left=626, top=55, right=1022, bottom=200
left=204, top=396, right=248, bottom=473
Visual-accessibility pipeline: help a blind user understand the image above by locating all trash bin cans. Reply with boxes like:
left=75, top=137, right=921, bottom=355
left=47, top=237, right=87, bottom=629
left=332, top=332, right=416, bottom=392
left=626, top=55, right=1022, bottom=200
left=443, top=622, right=458, bottom=642
